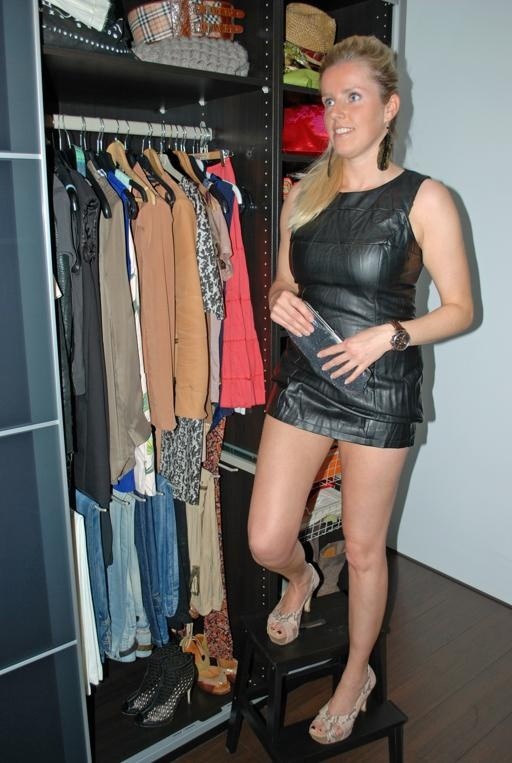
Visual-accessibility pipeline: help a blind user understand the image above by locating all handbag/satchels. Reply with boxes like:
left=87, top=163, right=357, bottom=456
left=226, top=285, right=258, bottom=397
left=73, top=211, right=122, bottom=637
left=286, top=301, right=371, bottom=396
left=40, top=0, right=250, bottom=77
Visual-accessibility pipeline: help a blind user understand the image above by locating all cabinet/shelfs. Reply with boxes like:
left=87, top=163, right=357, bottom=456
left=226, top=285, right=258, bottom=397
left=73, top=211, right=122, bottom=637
left=0, top=1, right=397, bottom=761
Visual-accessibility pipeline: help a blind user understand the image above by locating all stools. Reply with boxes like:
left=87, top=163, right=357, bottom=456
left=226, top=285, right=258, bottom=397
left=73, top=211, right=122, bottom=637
left=225, top=590, right=406, bottom=762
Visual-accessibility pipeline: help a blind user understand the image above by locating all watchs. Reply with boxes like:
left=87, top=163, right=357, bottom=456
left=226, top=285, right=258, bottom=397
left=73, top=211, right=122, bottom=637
left=387, top=320, right=410, bottom=353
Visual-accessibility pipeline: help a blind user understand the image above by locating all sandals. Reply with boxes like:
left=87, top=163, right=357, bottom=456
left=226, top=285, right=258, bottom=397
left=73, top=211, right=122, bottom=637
left=180, top=634, right=239, bottom=696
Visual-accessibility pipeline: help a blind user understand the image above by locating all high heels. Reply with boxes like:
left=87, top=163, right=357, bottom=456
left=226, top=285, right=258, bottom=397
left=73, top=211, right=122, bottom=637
left=122, top=644, right=198, bottom=729
left=309, top=665, right=377, bottom=745
left=267, top=563, right=320, bottom=646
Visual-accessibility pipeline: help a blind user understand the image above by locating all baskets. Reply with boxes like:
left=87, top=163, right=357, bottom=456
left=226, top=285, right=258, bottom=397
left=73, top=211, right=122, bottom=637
left=313, top=450, right=343, bottom=489
left=299, top=500, right=342, bottom=543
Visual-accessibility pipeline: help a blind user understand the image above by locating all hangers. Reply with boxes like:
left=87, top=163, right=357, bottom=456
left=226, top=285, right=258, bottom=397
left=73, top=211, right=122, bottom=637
left=45, top=111, right=254, bottom=219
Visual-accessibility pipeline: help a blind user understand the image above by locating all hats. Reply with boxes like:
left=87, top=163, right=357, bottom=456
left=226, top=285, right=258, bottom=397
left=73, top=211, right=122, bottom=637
left=286, top=3, right=336, bottom=66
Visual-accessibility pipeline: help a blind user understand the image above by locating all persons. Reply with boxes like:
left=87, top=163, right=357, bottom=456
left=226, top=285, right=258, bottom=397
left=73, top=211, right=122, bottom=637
left=245, top=37, right=478, bottom=743
